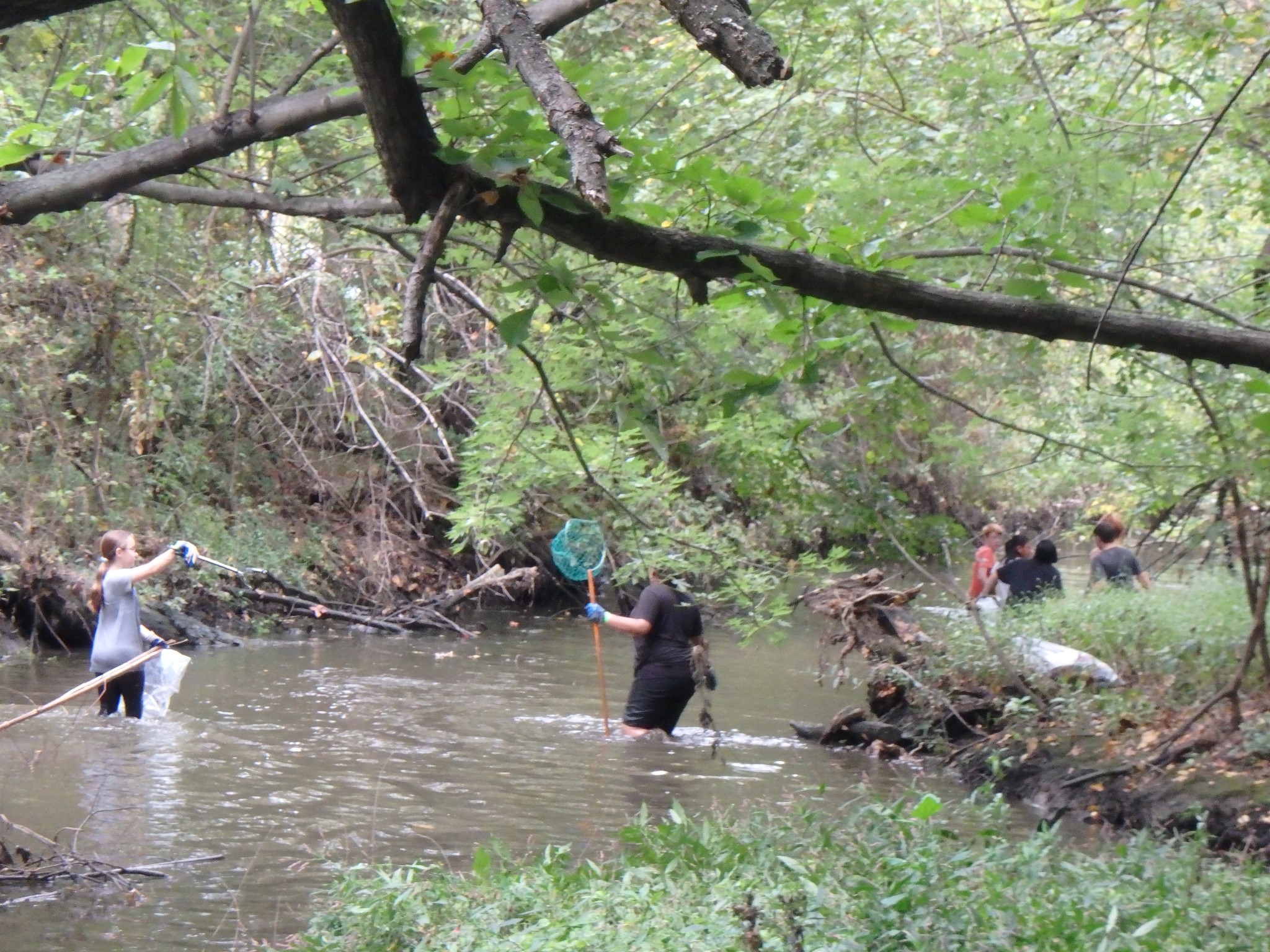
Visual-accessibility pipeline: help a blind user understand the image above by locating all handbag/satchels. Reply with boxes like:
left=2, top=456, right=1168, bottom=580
left=115, top=647, right=192, bottom=719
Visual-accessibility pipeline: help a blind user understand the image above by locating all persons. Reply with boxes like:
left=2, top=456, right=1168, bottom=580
left=84, top=530, right=200, bottom=720
left=586, top=548, right=717, bottom=740
left=966, top=514, right=1150, bottom=611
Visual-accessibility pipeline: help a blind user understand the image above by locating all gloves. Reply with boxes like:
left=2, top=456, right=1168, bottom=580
left=175, top=540, right=199, bottom=567
left=584, top=603, right=610, bottom=624
left=148, top=630, right=167, bottom=650
